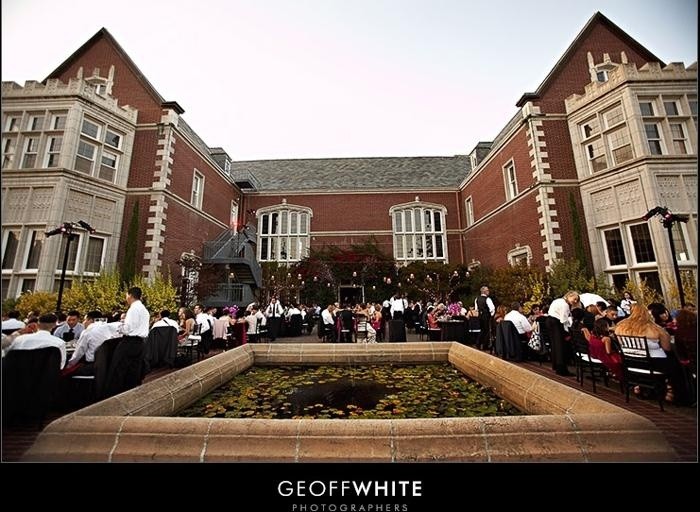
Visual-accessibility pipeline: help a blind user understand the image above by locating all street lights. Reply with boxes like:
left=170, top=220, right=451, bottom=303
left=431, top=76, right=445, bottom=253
left=174, top=255, right=205, bottom=310
left=47, top=221, right=94, bottom=315
left=639, top=204, right=690, bottom=308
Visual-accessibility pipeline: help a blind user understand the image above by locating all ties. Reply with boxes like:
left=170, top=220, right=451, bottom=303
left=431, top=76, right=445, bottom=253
left=273, top=304, right=275, bottom=318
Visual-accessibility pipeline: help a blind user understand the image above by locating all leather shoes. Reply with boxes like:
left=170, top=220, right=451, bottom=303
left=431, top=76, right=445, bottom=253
left=555, top=367, right=576, bottom=377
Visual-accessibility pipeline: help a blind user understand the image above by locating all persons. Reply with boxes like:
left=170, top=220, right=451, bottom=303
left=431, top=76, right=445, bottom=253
left=248, top=292, right=455, bottom=343
left=0, top=287, right=248, bottom=390
left=455, top=286, right=699, bottom=408
